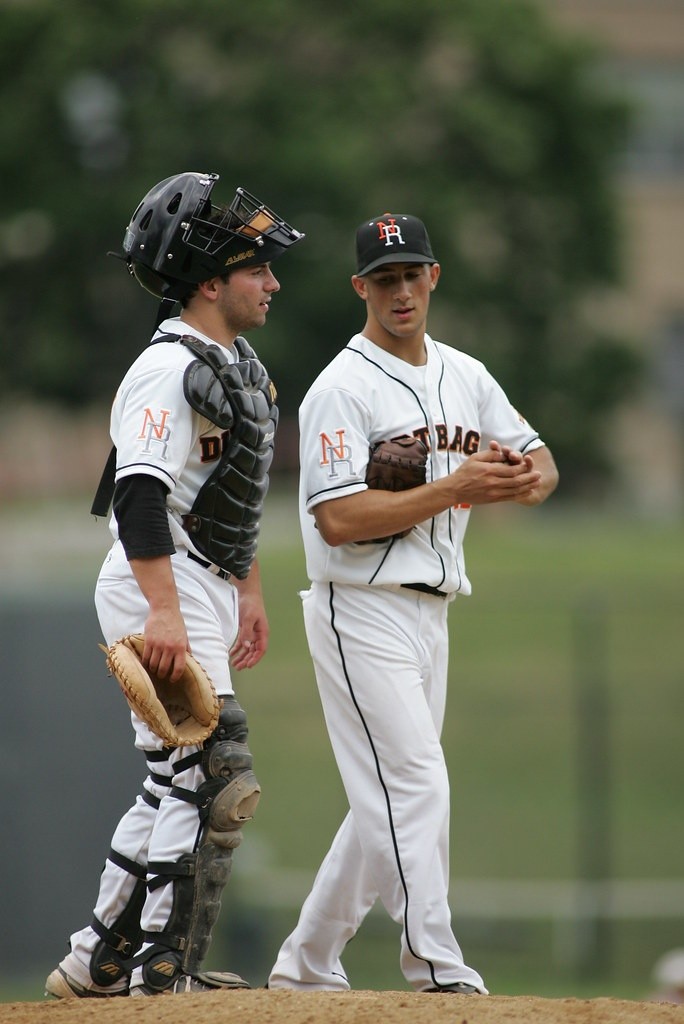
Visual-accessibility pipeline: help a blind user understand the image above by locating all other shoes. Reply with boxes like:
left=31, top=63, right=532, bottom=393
left=427, top=982, right=477, bottom=994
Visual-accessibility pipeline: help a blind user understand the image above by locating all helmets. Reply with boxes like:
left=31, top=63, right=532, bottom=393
left=107, top=172, right=305, bottom=302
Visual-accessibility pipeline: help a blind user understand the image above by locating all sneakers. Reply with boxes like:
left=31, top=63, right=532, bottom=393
left=129, top=974, right=251, bottom=998
left=46, top=951, right=130, bottom=998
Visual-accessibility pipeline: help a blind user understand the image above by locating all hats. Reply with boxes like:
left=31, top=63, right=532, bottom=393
left=223, top=207, right=291, bottom=273
left=355, top=213, right=438, bottom=277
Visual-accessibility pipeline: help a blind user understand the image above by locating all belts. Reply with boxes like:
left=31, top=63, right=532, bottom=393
left=400, top=583, right=447, bottom=598
left=187, top=550, right=232, bottom=580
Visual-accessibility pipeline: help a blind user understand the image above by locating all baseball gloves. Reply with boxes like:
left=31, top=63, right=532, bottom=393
left=313, top=433, right=429, bottom=545
left=98, top=631, right=224, bottom=750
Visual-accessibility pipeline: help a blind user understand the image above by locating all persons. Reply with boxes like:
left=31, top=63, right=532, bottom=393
left=268, top=214, right=558, bottom=995
left=44, top=172, right=304, bottom=1001
